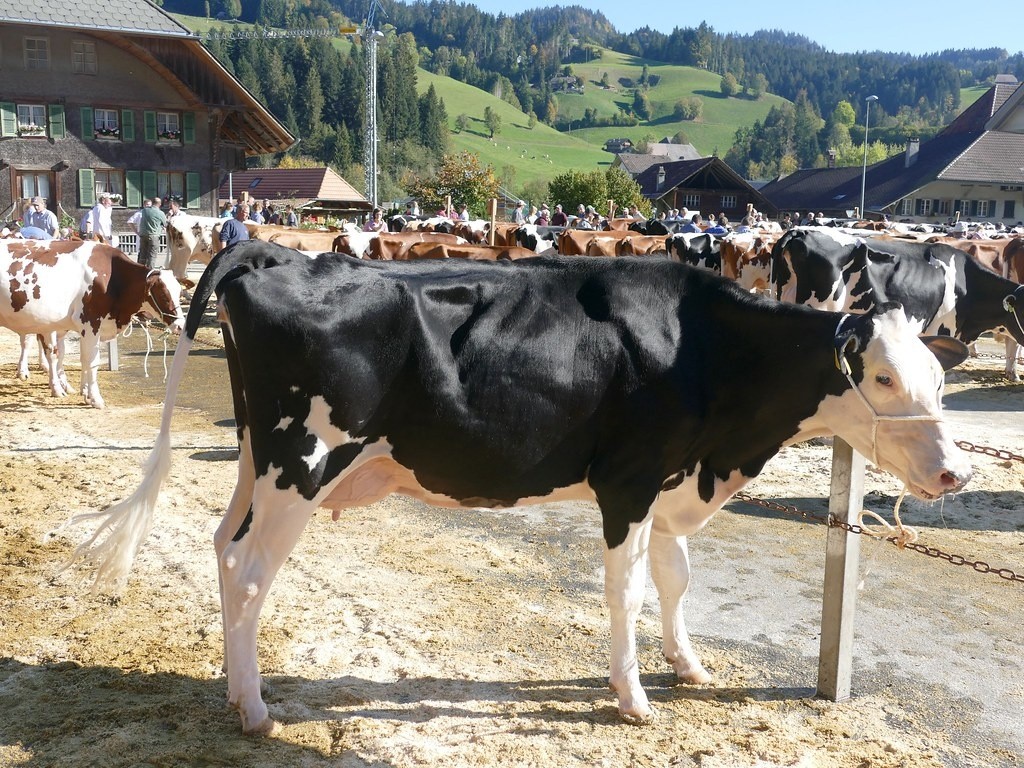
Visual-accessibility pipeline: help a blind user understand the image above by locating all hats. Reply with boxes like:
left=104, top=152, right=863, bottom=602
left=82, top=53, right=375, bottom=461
left=517, top=201, right=527, bottom=206
left=585, top=205, right=599, bottom=215
left=953, top=221, right=970, bottom=232
left=31, top=196, right=43, bottom=206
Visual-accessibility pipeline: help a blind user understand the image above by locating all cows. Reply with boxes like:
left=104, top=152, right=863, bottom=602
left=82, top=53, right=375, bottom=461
left=165, top=216, right=1024, bottom=294
left=51, top=239, right=975, bottom=741
left=768, top=225, right=1024, bottom=349
left=0, top=238, right=187, bottom=411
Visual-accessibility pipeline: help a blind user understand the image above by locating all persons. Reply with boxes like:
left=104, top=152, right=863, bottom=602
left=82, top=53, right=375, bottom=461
left=22, top=195, right=61, bottom=240
left=511, top=199, right=824, bottom=234
left=434, top=202, right=469, bottom=221
left=127, top=195, right=195, bottom=280
left=218, top=204, right=250, bottom=250
left=363, top=208, right=388, bottom=233
left=80, top=193, right=113, bottom=246
left=402, top=200, right=421, bottom=216
left=220, top=198, right=298, bottom=226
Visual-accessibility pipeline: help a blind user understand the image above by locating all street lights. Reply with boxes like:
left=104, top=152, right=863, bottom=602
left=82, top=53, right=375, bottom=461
left=370, top=30, right=384, bottom=210
left=859, top=95, right=879, bottom=219
